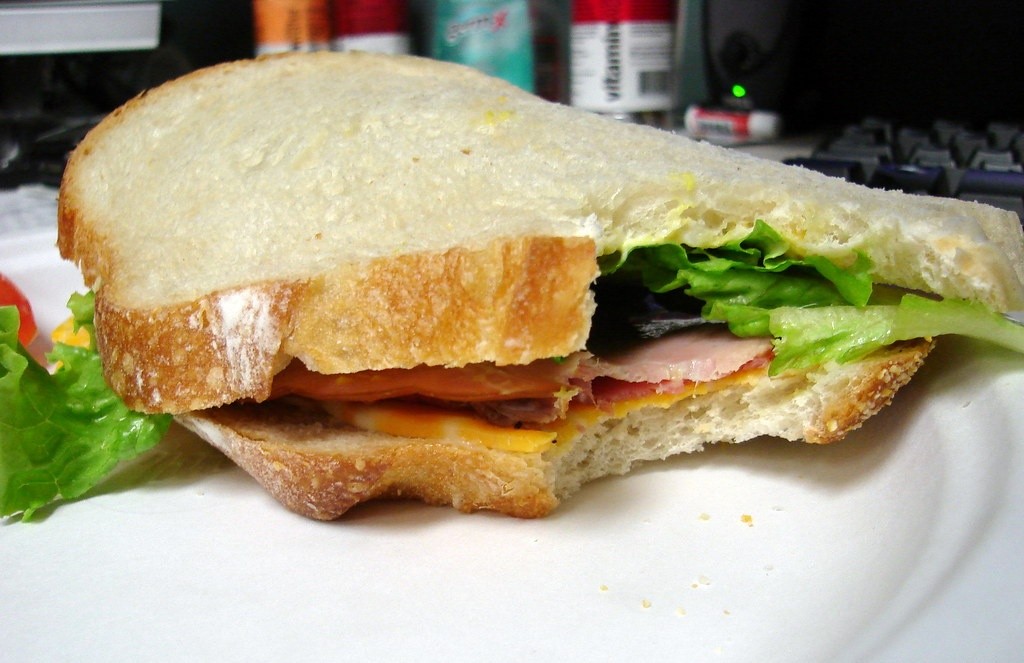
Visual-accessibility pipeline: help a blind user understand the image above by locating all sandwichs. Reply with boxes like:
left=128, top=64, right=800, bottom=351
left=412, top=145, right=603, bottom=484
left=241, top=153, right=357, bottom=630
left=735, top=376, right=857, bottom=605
left=0, top=51, right=1024, bottom=522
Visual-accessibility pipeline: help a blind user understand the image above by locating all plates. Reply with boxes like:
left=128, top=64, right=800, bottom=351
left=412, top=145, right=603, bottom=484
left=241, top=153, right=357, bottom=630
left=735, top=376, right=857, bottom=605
left=0, top=226, right=1024, bottom=663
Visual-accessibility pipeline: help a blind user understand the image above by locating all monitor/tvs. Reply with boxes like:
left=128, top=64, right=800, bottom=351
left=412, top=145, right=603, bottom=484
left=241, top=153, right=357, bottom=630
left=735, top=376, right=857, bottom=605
left=674, top=0, right=1024, bottom=141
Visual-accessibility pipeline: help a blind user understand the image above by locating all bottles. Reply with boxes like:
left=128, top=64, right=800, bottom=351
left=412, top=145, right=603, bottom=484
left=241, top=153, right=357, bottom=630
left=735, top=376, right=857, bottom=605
left=568, top=0, right=675, bottom=132
left=433, top=0, right=535, bottom=92
left=330, top=0, right=410, bottom=55
left=253, top=0, right=329, bottom=58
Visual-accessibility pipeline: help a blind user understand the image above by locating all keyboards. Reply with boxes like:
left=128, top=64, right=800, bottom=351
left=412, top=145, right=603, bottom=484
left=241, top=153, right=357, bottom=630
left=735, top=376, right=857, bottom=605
left=785, top=117, right=1024, bottom=222
